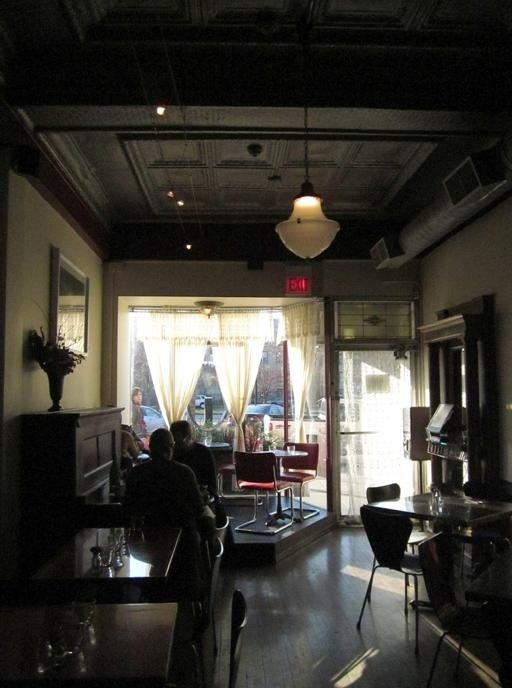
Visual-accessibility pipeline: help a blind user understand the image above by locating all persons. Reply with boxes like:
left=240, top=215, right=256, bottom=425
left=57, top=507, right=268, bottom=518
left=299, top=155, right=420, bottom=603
left=131, top=387, right=147, bottom=433
left=122, top=429, right=203, bottom=526
left=170, top=421, right=217, bottom=495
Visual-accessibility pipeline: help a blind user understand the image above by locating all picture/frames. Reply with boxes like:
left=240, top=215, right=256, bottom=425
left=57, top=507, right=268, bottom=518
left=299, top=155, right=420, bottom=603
left=49, top=243, right=93, bottom=361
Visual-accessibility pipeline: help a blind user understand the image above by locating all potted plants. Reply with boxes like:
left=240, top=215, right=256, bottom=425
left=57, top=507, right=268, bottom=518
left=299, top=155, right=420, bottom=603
left=31, top=330, right=79, bottom=414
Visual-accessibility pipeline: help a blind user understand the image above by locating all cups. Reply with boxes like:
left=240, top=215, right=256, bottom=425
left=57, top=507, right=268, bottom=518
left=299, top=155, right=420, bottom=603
left=257, top=444, right=263, bottom=452
left=287, top=446, right=295, bottom=455
left=24, top=596, right=98, bottom=672
left=269, top=445, right=275, bottom=454
left=99, top=514, right=146, bottom=568
left=428, top=486, right=443, bottom=505
left=429, top=504, right=442, bottom=517
left=205, top=436, right=212, bottom=447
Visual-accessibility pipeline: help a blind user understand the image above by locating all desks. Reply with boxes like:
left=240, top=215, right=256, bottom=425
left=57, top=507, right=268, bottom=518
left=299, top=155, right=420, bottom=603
left=1, top=597, right=186, bottom=684
left=19, top=520, right=184, bottom=590
left=362, top=492, right=512, bottom=613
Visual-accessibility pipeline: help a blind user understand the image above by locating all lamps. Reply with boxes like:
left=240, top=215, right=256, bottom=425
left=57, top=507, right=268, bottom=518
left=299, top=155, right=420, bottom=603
left=193, top=300, right=225, bottom=319
left=272, top=17, right=343, bottom=260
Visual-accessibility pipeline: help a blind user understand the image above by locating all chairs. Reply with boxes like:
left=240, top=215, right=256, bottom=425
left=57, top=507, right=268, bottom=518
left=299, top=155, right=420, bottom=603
left=461, top=472, right=512, bottom=556
left=364, top=479, right=430, bottom=612
left=415, top=532, right=510, bottom=687
left=231, top=447, right=296, bottom=537
left=76, top=502, right=125, bottom=527
left=277, top=441, right=321, bottom=525
left=216, top=436, right=263, bottom=507
left=169, top=509, right=252, bottom=687
left=353, top=505, right=446, bottom=657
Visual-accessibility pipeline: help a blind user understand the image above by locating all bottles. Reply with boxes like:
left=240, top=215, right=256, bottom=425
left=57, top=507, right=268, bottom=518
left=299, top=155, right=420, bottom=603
left=109, top=453, right=121, bottom=497
